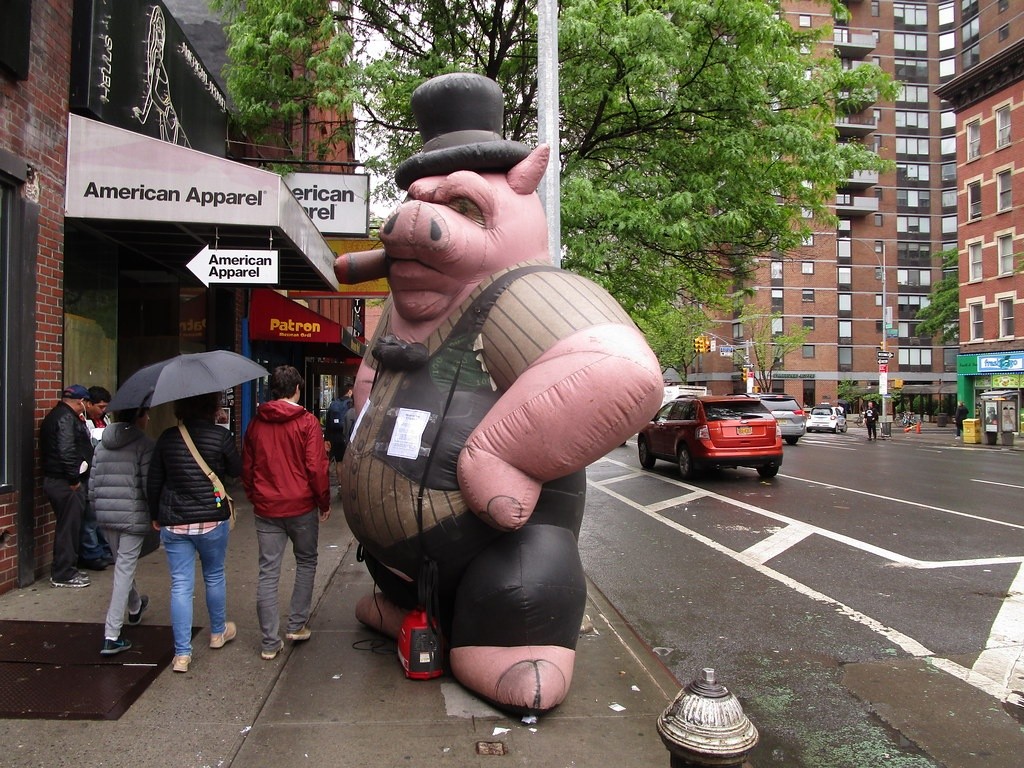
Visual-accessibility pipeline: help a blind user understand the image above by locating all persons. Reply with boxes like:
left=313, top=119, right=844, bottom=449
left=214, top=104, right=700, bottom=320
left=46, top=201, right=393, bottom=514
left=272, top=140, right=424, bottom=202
left=955, top=402, right=969, bottom=440
left=240, top=365, right=332, bottom=660
left=833, top=399, right=848, bottom=404
left=88, top=408, right=155, bottom=653
left=837, top=403, right=846, bottom=418
left=863, top=401, right=878, bottom=440
left=147, top=391, right=242, bottom=672
left=38, top=384, right=94, bottom=588
left=83, top=386, right=112, bottom=442
left=324, top=383, right=356, bottom=495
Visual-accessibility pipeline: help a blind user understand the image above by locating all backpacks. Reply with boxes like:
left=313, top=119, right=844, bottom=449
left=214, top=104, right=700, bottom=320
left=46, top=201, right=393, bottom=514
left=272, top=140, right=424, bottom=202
left=327, top=398, right=351, bottom=434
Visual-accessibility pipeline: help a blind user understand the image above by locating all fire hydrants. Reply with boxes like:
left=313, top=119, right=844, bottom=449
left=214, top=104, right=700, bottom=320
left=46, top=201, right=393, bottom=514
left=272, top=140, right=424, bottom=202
left=654, top=668, right=760, bottom=768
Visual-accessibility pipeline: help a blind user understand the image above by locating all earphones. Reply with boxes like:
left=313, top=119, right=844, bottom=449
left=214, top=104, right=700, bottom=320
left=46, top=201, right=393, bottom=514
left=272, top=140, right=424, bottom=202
left=81, top=402, right=84, bottom=408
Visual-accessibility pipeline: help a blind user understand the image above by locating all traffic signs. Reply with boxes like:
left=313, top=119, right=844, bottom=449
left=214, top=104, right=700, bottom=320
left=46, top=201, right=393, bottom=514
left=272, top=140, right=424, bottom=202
left=183, top=243, right=281, bottom=286
left=877, top=351, right=894, bottom=359
left=877, top=359, right=889, bottom=364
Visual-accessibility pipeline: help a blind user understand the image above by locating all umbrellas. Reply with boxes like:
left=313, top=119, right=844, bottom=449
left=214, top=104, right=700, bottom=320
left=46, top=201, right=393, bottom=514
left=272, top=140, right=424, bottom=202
left=103, top=350, right=272, bottom=413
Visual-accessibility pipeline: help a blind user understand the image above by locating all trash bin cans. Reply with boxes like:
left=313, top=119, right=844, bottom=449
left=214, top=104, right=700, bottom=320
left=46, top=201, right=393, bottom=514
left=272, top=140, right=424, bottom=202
left=937, top=413, right=948, bottom=427
left=962, top=418, right=982, bottom=444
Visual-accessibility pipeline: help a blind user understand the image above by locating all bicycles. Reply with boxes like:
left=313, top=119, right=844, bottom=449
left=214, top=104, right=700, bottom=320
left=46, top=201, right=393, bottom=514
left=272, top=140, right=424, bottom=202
left=854, top=415, right=867, bottom=427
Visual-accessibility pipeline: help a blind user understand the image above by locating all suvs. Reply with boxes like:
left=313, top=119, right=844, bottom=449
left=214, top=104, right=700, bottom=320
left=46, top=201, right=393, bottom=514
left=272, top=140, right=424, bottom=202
left=639, top=394, right=785, bottom=478
left=724, top=393, right=807, bottom=445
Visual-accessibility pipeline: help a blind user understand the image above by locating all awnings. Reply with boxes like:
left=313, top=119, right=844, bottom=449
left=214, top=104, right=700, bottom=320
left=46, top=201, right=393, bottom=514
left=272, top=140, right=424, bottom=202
left=249, top=288, right=367, bottom=358
left=64, top=112, right=339, bottom=292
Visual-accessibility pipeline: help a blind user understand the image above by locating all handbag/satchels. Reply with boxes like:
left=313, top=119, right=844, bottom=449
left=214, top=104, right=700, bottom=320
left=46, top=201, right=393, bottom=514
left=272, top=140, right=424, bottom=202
left=225, top=494, right=236, bottom=531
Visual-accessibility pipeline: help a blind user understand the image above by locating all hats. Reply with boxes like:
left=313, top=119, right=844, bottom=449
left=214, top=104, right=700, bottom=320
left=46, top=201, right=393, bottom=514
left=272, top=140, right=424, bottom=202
left=61, top=384, right=89, bottom=399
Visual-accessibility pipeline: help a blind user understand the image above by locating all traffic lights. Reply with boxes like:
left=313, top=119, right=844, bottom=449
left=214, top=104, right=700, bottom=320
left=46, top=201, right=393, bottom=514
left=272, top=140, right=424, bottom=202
left=742, top=367, right=749, bottom=382
left=880, top=341, right=889, bottom=351
left=698, top=336, right=704, bottom=353
left=693, top=336, right=700, bottom=353
left=755, top=386, right=760, bottom=393
left=703, top=337, right=711, bottom=354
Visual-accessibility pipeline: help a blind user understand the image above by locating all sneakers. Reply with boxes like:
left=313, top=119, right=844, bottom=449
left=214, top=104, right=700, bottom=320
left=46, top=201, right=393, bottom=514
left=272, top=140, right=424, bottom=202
left=209, top=622, right=236, bottom=648
left=128, top=595, right=149, bottom=625
left=261, top=639, right=284, bottom=660
left=101, top=637, right=131, bottom=654
left=49, top=572, right=91, bottom=587
left=172, top=656, right=192, bottom=672
left=285, top=628, right=312, bottom=640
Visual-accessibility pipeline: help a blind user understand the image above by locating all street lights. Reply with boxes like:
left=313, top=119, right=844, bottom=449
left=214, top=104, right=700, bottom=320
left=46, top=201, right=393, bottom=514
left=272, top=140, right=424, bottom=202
left=838, top=237, right=889, bottom=438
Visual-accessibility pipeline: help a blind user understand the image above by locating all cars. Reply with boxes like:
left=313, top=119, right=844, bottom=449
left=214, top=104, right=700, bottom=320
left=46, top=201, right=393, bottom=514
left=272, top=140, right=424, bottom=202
left=807, top=406, right=848, bottom=433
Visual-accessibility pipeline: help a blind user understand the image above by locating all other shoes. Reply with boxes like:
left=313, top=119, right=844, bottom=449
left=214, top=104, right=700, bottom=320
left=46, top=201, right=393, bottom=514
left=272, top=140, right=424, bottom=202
left=955, top=436, right=960, bottom=440
left=77, top=560, right=107, bottom=570
